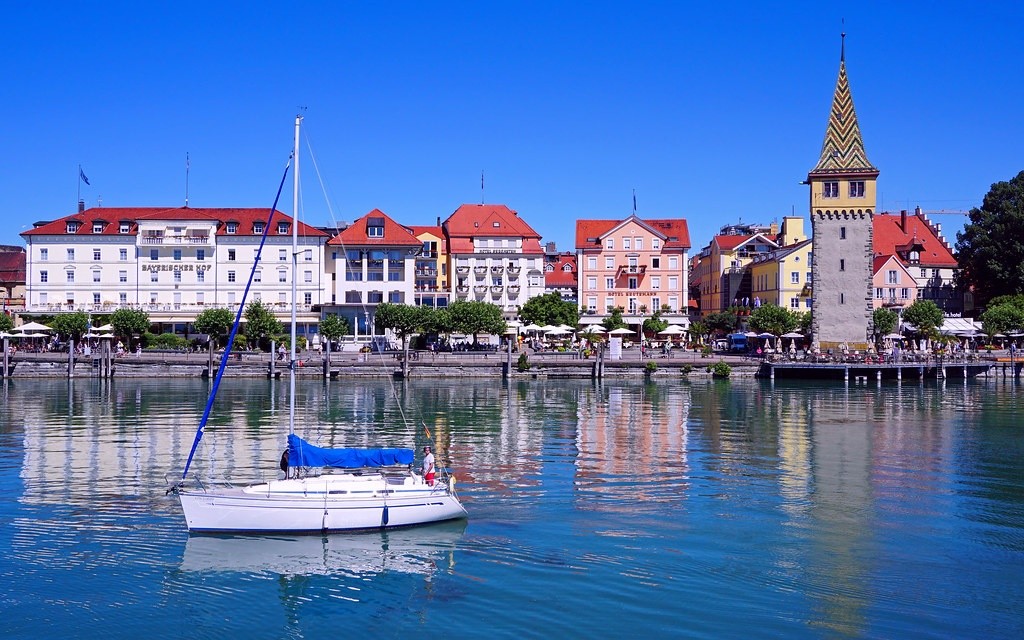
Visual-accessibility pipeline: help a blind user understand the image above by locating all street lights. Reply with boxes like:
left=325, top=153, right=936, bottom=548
left=86, top=308, right=93, bottom=347
left=364, top=311, right=369, bottom=363
left=639, top=313, right=648, bottom=362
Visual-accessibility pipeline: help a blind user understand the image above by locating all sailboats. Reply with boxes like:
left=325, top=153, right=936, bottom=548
left=163, top=102, right=469, bottom=531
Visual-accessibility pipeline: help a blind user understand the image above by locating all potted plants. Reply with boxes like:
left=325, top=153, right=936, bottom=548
left=584, top=350, right=590, bottom=359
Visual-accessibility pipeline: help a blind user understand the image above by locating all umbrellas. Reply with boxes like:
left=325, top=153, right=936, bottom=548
left=975, top=333, right=1024, bottom=337
left=842, top=338, right=850, bottom=353
left=744, top=331, right=805, bottom=338
left=868, top=338, right=874, bottom=349
left=0, top=332, right=50, bottom=345
left=90, top=323, right=116, bottom=331
left=523, top=323, right=636, bottom=334
left=11, top=320, right=53, bottom=332
left=911, top=337, right=969, bottom=350
left=883, top=332, right=906, bottom=349
left=814, top=337, right=821, bottom=354
left=83, top=333, right=116, bottom=338
left=658, top=324, right=690, bottom=334
left=764, top=338, right=797, bottom=349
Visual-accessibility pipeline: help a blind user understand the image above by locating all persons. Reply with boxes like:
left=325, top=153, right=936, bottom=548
left=116, top=340, right=128, bottom=352
left=136, top=342, right=141, bottom=358
left=280, top=449, right=289, bottom=479
left=756, top=346, right=762, bottom=358
left=423, top=446, right=435, bottom=487
left=77, top=340, right=97, bottom=353
left=276, top=343, right=286, bottom=363
left=640, top=340, right=645, bottom=356
